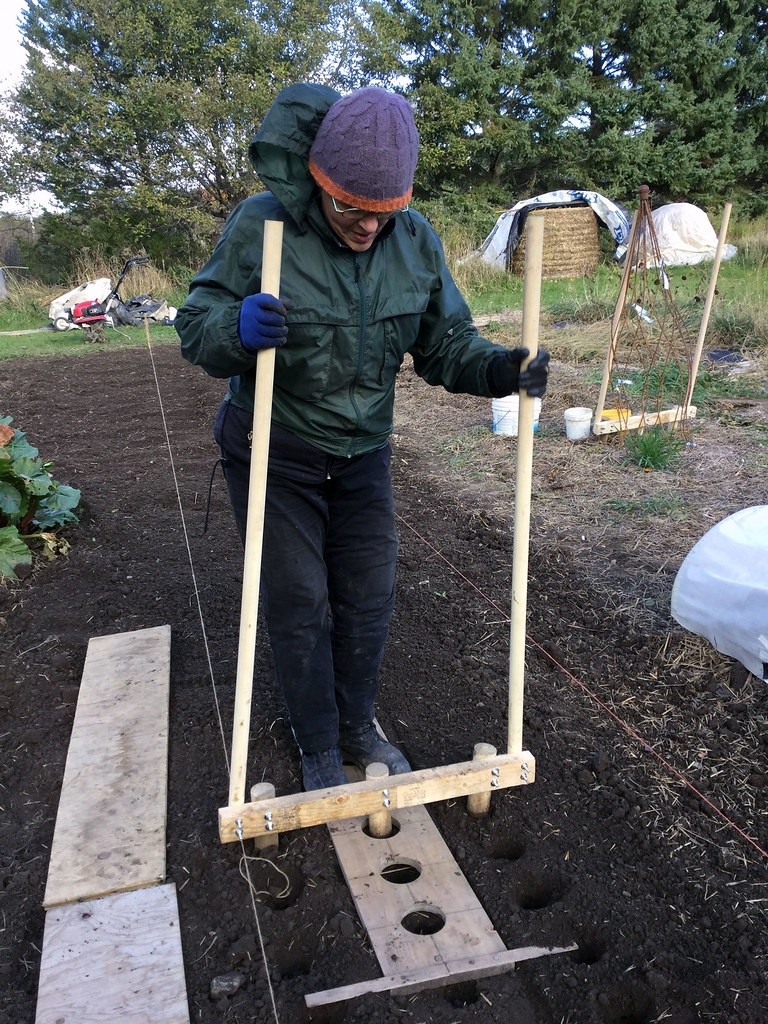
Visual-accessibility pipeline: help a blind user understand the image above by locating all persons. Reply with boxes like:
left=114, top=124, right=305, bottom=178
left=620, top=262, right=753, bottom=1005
left=172, top=78, right=551, bottom=799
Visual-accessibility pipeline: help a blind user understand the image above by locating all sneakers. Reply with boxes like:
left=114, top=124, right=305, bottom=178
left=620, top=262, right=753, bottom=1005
left=302, top=746, right=350, bottom=791
left=339, top=721, right=412, bottom=777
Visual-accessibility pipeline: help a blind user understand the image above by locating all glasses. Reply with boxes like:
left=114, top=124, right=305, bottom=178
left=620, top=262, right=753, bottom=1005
left=333, top=197, right=409, bottom=221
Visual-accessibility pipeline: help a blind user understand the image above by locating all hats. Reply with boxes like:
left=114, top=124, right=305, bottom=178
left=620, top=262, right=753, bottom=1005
left=309, top=87, right=419, bottom=211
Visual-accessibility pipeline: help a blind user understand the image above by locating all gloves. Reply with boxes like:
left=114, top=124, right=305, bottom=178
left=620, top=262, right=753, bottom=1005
left=488, top=346, right=549, bottom=399
left=236, top=293, right=296, bottom=352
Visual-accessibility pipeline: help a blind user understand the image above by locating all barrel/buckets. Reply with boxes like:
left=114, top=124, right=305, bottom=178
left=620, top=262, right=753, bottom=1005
left=491, top=395, right=542, bottom=437
left=565, top=407, right=593, bottom=440
